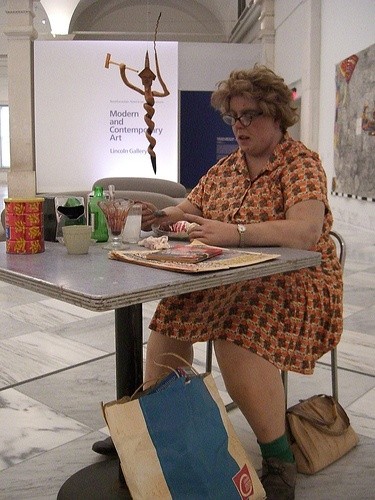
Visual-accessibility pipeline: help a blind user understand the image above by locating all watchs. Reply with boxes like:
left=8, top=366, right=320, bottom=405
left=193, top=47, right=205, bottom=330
left=236, top=223, right=246, bottom=249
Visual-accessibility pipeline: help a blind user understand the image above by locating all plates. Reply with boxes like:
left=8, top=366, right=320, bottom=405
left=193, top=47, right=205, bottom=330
left=150, top=222, right=189, bottom=240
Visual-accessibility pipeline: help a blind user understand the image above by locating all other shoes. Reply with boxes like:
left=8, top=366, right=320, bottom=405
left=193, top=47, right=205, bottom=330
left=92, top=436, right=117, bottom=456
left=260, top=452, right=298, bottom=500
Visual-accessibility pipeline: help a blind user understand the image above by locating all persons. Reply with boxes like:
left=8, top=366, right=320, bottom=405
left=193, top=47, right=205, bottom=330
left=92, top=66, right=342, bottom=500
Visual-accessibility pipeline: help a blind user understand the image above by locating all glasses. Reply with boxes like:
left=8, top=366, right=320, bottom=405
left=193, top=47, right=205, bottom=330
left=222, top=109, right=265, bottom=127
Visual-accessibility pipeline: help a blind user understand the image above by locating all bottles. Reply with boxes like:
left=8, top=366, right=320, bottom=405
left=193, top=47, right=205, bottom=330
left=88, top=185, right=110, bottom=242
left=105, top=184, right=126, bottom=236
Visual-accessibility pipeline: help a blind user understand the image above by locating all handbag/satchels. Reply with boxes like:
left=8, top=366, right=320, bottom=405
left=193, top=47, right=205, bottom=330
left=99, top=352, right=267, bottom=500
left=286, top=393, right=359, bottom=475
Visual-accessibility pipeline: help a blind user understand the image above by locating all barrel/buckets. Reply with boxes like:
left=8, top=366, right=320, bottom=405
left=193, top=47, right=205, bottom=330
left=4, top=197, right=47, bottom=255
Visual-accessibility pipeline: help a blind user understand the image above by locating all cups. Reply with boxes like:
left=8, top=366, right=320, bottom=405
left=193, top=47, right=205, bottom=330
left=62, top=225, right=93, bottom=256
left=122, top=203, right=144, bottom=243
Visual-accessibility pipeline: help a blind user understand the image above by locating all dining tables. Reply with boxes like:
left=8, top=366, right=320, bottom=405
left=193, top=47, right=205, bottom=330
left=0, top=240, right=322, bottom=500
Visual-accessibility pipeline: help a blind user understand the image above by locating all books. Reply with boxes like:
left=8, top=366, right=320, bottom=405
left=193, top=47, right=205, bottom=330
left=147, top=243, right=223, bottom=264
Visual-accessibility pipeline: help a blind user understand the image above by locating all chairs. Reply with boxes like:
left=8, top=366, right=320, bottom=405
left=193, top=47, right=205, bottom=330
left=91, top=177, right=187, bottom=210
left=205, top=231, right=346, bottom=412
